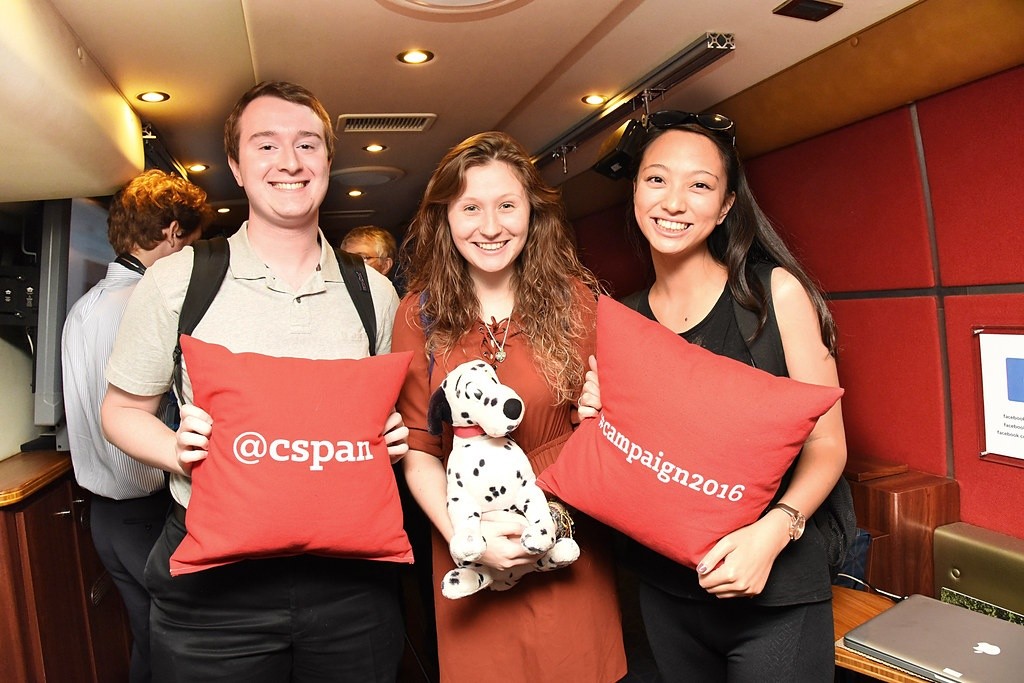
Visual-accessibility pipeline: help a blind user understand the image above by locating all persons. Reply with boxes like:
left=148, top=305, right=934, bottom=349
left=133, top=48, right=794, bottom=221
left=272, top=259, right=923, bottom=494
left=340, top=226, right=403, bottom=301
left=391, top=131, right=629, bottom=683
left=62, top=170, right=212, bottom=683
left=578, top=125, right=847, bottom=683
left=100, top=80, right=410, bottom=683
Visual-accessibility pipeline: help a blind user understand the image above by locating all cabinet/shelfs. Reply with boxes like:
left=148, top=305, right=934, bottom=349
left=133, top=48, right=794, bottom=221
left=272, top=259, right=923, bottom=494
left=0, top=467, right=135, bottom=683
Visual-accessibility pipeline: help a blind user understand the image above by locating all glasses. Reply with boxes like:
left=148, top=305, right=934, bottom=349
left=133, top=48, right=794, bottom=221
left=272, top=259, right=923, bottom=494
left=357, top=252, right=382, bottom=263
left=640, top=110, right=736, bottom=151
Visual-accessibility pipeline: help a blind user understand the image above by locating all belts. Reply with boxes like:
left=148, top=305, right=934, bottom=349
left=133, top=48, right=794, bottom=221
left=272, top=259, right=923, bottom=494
left=172, top=499, right=186, bottom=528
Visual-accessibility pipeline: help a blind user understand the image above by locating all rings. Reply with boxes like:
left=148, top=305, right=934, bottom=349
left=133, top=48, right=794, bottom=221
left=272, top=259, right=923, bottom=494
left=578, top=397, right=581, bottom=406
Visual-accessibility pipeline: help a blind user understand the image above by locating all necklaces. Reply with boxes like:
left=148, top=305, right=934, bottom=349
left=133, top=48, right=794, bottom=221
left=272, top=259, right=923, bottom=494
left=479, top=309, right=511, bottom=363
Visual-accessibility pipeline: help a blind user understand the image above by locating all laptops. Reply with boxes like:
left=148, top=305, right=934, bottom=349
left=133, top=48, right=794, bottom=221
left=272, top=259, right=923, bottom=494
left=844, top=594, right=1024, bottom=683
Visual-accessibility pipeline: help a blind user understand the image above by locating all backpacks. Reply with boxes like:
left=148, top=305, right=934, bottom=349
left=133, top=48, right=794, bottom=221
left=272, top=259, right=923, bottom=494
left=618, top=256, right=854, bottom=573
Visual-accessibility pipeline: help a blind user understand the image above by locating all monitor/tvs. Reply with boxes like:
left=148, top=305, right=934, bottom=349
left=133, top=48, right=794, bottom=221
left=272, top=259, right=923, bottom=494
left=34, top=198, right=119, bottom=452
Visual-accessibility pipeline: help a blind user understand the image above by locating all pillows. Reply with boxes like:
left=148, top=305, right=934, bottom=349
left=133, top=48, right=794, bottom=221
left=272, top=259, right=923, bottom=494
left=168, top=327, right=417, bottom=575
left=534, top=292, right=845, bottom=585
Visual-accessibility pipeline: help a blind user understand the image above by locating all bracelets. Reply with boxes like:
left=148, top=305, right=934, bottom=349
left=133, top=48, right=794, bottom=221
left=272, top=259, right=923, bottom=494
left=547, top=497, right=575, bottom=540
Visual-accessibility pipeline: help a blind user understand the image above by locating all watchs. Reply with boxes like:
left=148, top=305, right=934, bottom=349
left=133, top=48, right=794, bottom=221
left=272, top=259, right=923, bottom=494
left=770, top=503, right=805, bottom=540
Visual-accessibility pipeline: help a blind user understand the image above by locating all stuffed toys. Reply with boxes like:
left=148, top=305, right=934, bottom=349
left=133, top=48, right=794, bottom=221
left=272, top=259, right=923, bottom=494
left=427, top=360, right=580, bottom=600
